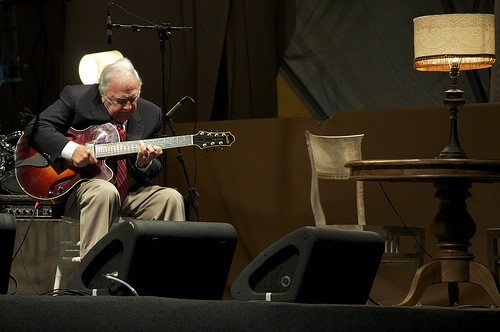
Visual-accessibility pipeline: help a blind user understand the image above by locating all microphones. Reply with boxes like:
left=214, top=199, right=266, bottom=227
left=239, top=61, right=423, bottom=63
left=106, top=5, right=112, bottom=45
left=166, top=96, right=187, bottom=118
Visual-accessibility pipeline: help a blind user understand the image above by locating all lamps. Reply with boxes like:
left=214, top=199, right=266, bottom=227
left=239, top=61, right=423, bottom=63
left=414, top=14, right=496, bottom=159
left=79, top=50, right=124, bottom=84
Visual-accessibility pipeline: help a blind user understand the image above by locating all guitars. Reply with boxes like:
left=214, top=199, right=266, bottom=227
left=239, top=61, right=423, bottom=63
left=14, top=123, right=235, bottom=205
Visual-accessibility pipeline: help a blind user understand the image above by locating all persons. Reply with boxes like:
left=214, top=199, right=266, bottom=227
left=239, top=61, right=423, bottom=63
left=24, top=58, right=185, bottom=259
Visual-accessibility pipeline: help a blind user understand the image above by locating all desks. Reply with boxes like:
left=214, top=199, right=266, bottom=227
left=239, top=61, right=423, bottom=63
left=343, top=159, right=500, bottom=307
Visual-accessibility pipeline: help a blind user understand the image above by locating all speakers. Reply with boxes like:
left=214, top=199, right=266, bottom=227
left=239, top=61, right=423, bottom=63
left=232, top=226, right=385, bottom=305
left=69, top=220, right=238, bottom=301
left=0, top=213, right=15, bottom=297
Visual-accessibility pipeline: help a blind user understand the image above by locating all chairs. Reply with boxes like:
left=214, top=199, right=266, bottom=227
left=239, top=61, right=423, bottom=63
left=54, top=213, right=99, bottom=294
left=304, top=129, right=425, bottom=306
